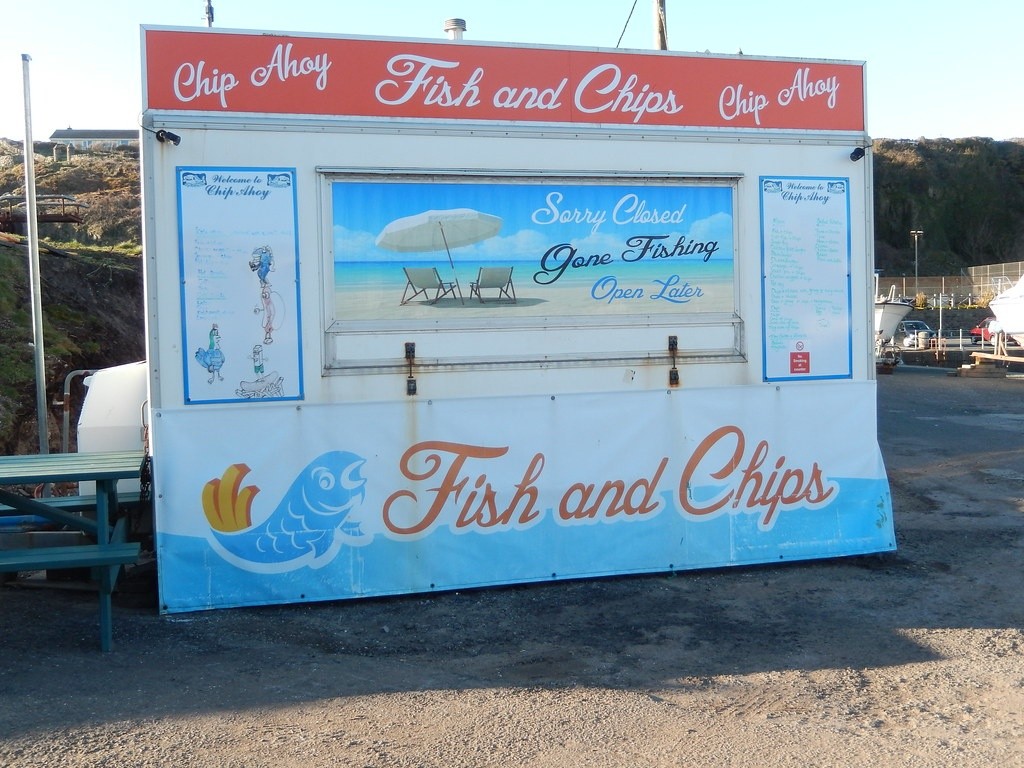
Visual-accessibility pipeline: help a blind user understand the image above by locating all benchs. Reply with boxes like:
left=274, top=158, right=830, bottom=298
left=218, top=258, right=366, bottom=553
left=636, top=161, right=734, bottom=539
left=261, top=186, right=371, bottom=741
left=0, top=541, right=142, bottom=573
left=0, top=490, right=152, bottom=517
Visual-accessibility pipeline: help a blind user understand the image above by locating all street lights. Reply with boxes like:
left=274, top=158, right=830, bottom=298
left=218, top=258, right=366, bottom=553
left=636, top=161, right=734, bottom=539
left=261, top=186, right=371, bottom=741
left=910, top=230, right=924, bottom=297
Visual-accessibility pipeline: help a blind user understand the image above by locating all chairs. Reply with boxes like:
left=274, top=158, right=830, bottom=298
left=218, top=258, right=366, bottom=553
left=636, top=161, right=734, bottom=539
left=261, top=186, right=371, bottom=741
left=399, top=267, right=456, bottom=306
left=469, top=266, right=516, bottom=304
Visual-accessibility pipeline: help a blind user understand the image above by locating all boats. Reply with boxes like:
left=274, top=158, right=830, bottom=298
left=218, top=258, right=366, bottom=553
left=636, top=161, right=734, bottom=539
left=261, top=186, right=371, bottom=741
left=872, top=270, right=916, bottom=374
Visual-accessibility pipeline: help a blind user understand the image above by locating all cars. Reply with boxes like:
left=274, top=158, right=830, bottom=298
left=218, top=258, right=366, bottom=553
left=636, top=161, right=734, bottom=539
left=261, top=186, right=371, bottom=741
left=896, top=321, right=937, bottom=347
left=969, top=317, right=1019, bottom=346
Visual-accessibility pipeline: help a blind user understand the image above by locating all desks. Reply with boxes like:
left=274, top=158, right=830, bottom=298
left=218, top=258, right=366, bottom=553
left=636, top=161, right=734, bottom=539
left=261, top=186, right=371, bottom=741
left=0, top=449, right=147, bottom=653
